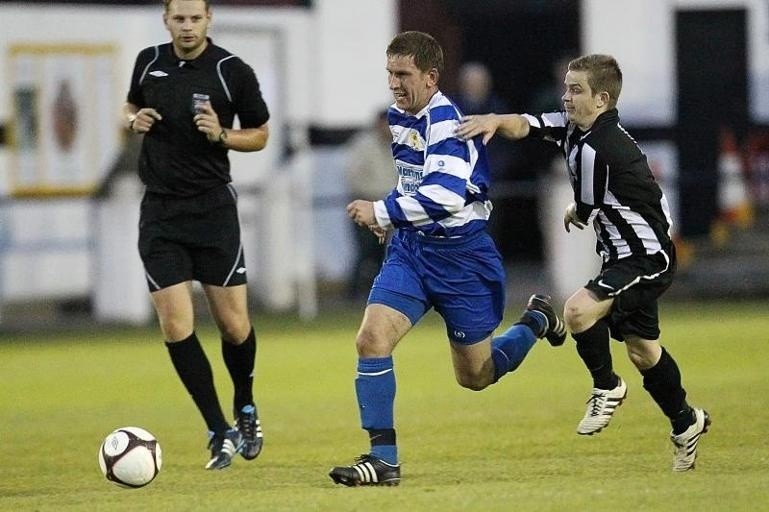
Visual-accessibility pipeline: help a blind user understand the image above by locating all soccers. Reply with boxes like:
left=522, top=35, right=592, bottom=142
left=99, top=427, right=163, bottom=489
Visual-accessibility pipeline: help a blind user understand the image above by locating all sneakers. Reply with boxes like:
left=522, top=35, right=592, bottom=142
left=575, top=372, right=628, bottom=437
left=204, top=426, right=249, bottom=471
left=668, top=404, right=713, bottom=474
left=232, top=400, right=264, bottom=462
left=522, top=291, right=567, bottom=347
left=327, top=453, right=402, bottom=488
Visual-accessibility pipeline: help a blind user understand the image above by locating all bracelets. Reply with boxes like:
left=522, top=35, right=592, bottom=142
left=218, top=126, right=228, bottom=146
left=129, top=117, right=136, bottom=133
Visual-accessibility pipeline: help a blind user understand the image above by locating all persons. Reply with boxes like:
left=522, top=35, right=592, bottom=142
left=119, top=0, right=274, bottom=474
left=453, top=50, right=710, bottom=475
left=329, top=29, right=567, bottom=487
left=454, top=59, right=506, bottom=166
left=339, top=108, right=398, bottom=308
